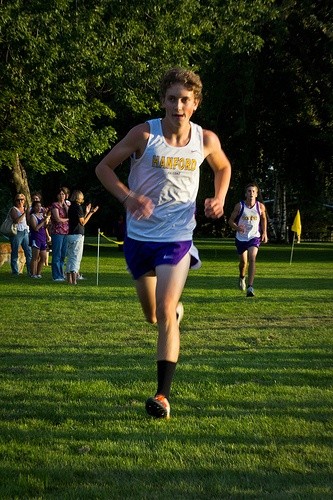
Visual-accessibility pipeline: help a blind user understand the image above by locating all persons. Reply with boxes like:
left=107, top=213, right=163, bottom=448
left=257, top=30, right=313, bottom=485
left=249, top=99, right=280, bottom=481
left=94, top=68, right=231, bottom=417
left=67, top=191, right=99, bottom=285
left=50, top=187, right=73, bottom=281
left=228, top=184, right=268, bottom=297
left=8, top=192, right=52, bottom=279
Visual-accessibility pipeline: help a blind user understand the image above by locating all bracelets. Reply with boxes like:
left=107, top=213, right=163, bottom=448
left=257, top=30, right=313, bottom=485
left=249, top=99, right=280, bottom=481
left=120, top=191, right=132, bottom=203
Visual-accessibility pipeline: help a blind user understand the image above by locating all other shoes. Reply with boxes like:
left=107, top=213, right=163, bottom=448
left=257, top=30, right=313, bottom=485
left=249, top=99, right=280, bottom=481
left=238, top=277, right=246, bottom=290
left=145, top=395, right=170, bottom=420
left=31, top=274, right=41, bottom=279
left=246, top=287, right=254, bottom=296
left=62, top=275, right=67, bottom=279
left=176, top=302, right=184, bottom=327
left=53, top=278, right=65, bottom=281
left=76, top=274, right=84, bottom=280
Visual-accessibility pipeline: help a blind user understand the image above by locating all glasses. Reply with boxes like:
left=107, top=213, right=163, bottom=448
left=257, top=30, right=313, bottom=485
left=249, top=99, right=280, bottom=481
left=17, top=199, right=24, bottom=201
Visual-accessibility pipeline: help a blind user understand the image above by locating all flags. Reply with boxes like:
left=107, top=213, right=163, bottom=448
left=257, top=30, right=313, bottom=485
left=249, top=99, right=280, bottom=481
left=291, top=210, right=302, bottom=237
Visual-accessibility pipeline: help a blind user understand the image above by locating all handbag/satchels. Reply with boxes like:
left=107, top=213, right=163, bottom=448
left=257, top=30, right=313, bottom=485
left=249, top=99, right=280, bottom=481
left=0, top=206, right=18, bottom=235
